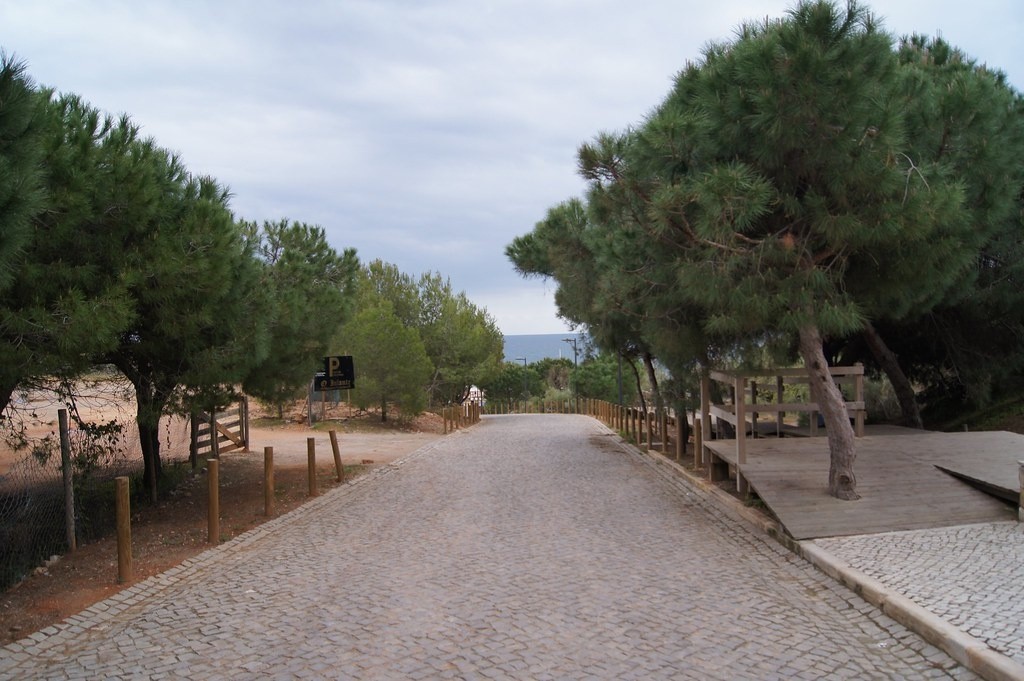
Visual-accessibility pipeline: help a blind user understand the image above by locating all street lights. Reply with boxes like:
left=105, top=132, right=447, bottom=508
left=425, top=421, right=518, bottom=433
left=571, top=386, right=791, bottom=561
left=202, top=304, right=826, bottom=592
left=562, top=338, right=577, bottom=399
left=516, top=357, right=527, bottom=412
